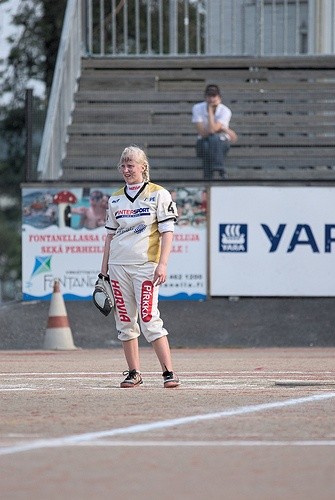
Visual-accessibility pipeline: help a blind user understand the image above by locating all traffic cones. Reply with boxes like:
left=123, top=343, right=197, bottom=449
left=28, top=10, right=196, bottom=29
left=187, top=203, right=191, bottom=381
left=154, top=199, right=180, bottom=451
left=42, top=280, right=81, bottom=351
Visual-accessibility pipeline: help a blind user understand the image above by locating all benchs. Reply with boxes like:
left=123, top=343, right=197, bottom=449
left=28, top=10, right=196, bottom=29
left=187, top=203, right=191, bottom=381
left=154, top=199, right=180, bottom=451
left=60, top=54, right=335, bottom=187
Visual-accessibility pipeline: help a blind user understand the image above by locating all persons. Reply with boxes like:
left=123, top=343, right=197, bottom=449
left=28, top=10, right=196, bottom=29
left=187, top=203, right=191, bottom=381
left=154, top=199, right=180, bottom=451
left=69, top=189, right=210, bottom=229
left=96, top=146, right=180, bottom=388
left=191, top=85, right=237, bottom=183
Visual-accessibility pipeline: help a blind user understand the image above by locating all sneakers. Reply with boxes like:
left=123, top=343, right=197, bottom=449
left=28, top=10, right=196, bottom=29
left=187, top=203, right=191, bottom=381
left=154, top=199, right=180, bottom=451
left=162, top=362, right=180, bottom=388
left=119, top=369, right=144, bottom=388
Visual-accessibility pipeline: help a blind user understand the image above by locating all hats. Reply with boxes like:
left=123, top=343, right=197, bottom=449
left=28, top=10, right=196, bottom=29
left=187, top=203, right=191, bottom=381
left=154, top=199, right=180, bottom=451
left=204, top=84, right=220, bottom=98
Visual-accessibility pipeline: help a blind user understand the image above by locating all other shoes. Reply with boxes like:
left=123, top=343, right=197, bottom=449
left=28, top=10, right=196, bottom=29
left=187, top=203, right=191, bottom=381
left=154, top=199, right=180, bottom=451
left=211, top=174, right=223, bottom=180
